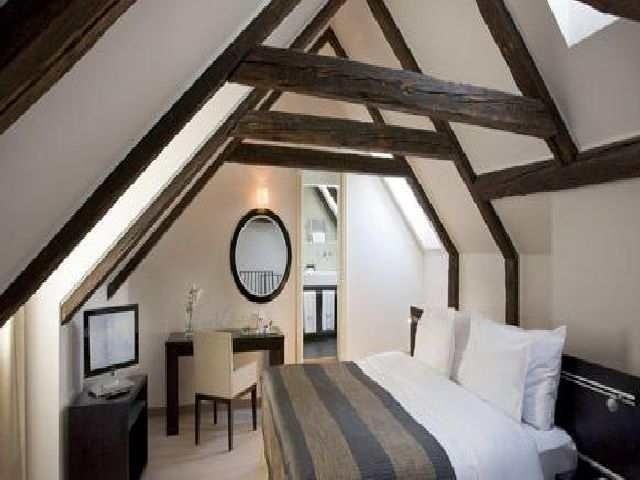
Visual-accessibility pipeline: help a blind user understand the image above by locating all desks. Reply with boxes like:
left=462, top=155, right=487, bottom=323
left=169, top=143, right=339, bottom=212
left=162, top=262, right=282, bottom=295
left=164, top=326, right=284, bottom=436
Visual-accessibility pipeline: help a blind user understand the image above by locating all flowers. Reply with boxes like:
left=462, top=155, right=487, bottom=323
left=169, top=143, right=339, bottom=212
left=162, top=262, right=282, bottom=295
left=182, top=283, right=202, bottom=313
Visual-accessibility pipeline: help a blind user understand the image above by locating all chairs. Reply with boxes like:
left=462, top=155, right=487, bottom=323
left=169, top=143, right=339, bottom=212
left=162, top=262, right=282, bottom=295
left=193, top=330, right=257, bottom=451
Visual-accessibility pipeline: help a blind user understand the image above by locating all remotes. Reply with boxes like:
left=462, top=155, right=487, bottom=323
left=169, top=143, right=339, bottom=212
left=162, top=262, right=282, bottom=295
left=105, top=388, right=131, bottom=399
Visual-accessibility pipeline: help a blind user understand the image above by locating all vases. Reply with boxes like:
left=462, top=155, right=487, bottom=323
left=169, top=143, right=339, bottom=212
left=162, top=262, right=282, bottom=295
left=186, top=313, right=193, bottom=337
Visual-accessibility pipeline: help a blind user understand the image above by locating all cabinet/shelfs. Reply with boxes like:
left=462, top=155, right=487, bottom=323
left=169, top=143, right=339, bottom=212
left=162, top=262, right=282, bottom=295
left=66, top=372, right=149, bottom=480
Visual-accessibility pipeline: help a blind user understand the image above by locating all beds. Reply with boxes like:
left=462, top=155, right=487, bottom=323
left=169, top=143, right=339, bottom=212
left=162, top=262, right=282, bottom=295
left=260, top=306, right=578, bottom=480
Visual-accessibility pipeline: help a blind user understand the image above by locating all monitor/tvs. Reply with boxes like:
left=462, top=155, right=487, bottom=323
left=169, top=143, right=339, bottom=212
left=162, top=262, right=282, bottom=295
left=84, top=303, right=140, bottom=397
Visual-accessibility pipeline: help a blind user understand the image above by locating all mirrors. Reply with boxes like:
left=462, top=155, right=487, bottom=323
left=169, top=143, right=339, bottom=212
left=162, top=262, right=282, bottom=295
left=230, top=207, right=292, bottom=303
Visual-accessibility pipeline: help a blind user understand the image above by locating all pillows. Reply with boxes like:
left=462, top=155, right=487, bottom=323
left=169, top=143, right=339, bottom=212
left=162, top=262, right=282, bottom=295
left=461, top=321, right=536, bottom=422
left=522, top=325, right=570, bottom=432
left=452, top=312, right=472, bottom=382
left=413, top=307, right=458, bottom=380
